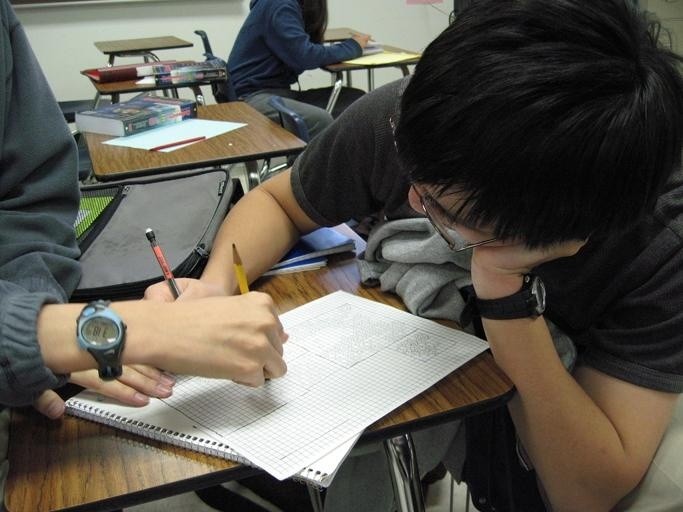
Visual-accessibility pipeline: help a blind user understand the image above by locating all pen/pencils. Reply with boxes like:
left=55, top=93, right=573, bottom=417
left=150, top=136, right=205, bottom=151
left=232, top=242, right=249, bottom=294
left=347, top=31, right=377, bottom=43
left=146, top=228, right=181, bottom=301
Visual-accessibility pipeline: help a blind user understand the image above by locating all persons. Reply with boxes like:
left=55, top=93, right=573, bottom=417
left=0, top=0, right=291, bottom=512
left=222, top=0, right=370, bottom=168
left=132, top=0, right=682, bottom=512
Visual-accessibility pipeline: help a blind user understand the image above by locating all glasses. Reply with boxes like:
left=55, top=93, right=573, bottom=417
left=390, top=115, right=511, bottom=252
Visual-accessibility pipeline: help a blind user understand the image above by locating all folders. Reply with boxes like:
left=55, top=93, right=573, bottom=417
left=79, top=60, right=175, bottom=83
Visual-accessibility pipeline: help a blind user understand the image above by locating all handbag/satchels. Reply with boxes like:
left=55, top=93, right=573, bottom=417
left=70, top=168, right=233, bottom=302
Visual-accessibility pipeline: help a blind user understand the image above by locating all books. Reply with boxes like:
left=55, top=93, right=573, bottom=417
left=260, top=223, right=357, bottom=278
left=63, top=366, right=367, bottom=493
left=323, top=40, right=384, bottom=57
left=74, top=91, right=198, bottom=136
left=79, top=57, right=229, bottom=87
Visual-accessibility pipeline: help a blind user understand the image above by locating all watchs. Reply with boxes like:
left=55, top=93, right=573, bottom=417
left=468, top=271, right=548, bottom=321
left=75, top=297, right=129, bottom=383
